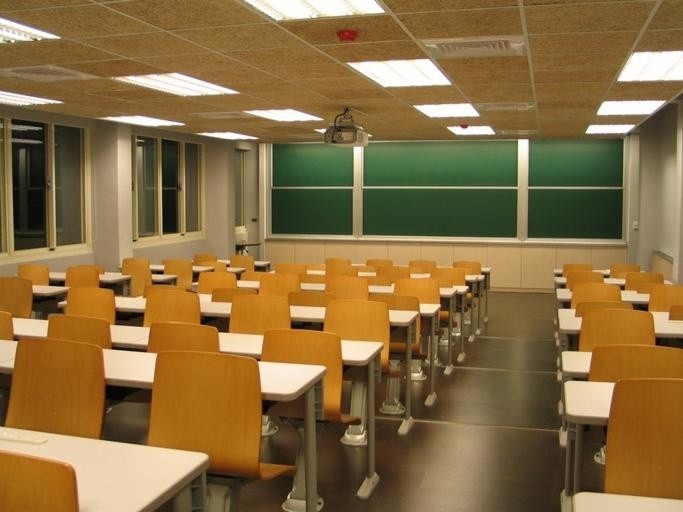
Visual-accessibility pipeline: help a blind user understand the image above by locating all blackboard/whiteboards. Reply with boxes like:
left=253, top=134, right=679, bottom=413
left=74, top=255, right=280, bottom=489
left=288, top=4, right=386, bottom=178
left=528, top=138, right=624, bottom=187
left=271, top=189, right=353, bottom=236
left=362, top=189, right=518, bottom=237
left=364, top=141, right=518, bottom=187
left=527, top=189, right=624, bottom=240
left=272, top=144, right=353, bottom=187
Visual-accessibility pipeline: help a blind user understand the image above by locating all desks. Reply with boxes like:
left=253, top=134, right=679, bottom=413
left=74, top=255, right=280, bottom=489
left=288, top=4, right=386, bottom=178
left=269, top=268, right=476, bottom=346
left=553, top=288, right=651, bottom=309
left=214, top=258, right=270, bottom=270
left=48, top=271, right=131, bottom=295
left=151, top=273, right=176, bottom=285
left=191, top=277, right=458, bottom=377
left=150, top=264, right=213, bottom=279
left=32, top=284, right=68, bottom=317
left=571, top=489, right=682, bottom=512
left=555, top=350, right=593, bottom=446
left=562, top=379, right=616, bottom=506
left=0, top=340, right=327, bottom=512
left=10, top=315, right=383, bottom=499
left=552, top=268, right=647, bottom=277
left=0, top=423, right=212, bottom=512
left=456, top=285, right=468, bottom=365
left=476, top=274, right=487, bottom=337
left=553, top=307, right=683, bottom=351
left=553, top=275, right=677, bottom=290
left=415, top=301, right=443, bottom=408
left=57, top=294, right=420, bottom=435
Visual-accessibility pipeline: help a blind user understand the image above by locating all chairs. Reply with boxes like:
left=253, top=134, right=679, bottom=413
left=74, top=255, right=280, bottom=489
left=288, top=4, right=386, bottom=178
left=1, top=450, right=79, bottom=512
left=286, top=292, right=335, bottom=307
left=367, top=257, right=436, bottom=284
left=258, top=328, right=359, bottom=512
left=141, top=288, right=202, bottom=329
left=666, top=306, right=682, bottom=320
left=269, top=256, right=361, bottom=284
left=227, top=295, right=292, bottom=336
left=562, top=263, right=595, bottom=278
left=1, top=276, right=33, bottom=320
left=578, top=308, right=656, bottom=354
left=146, top=321, right=224, bottom=355
left=574, top=301, right=632, bottom=317
left=623, top=272, right=664, bottom=290
left=323, top=275, right=370, bottom=301
left=121, top=257, right=152, bottom=278
left=323, top=299, right=406, bottom=417
left=163, top=259, right=194, bottom=287
left=570, top=283, right=621, bottom=311
left=1, top=310, right=13, bottom=341
left=44, top=314, right=110, bottom=351
left=432, top=273, right=454, bottom=352
left=190, top=254, right=256, bottom=273
left=209, top=287, right=258, bottom=304
left=452, top=259, right=482, bottom=325
left=367, top=292, right=426, bottom=381
left=258, top=274, right=300, bottom=300
left=604, top=376, right=683, bottom=501
left=144, top=350, right=295, bottom=512
left=241, top=272, right=270, bottom=282
left=196, top=272, right=240, bottom=294
left=63, top=287, right=117, bottom=324
left=17, top=263, right=52, bottom=285
left=434, top=268, right=465, bottom=338
left=63, top=264, right=99, bottom=289
left=587, top=345, right=681, bottom=383
left=645, top=283, right=682, bottom=311
left=393, top=278, right=441, bottom=367
left=3, top=337, right=106, bottom=443
left=609, top=262, right=641, bottom=280
left=129, top=270, right=154, bottom=299
left=565, top=271, right=606, bottom=290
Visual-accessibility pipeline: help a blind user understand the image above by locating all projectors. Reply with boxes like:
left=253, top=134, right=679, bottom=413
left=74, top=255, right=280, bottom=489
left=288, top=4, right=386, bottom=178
left=324, top=109, right=369, bottom=146
